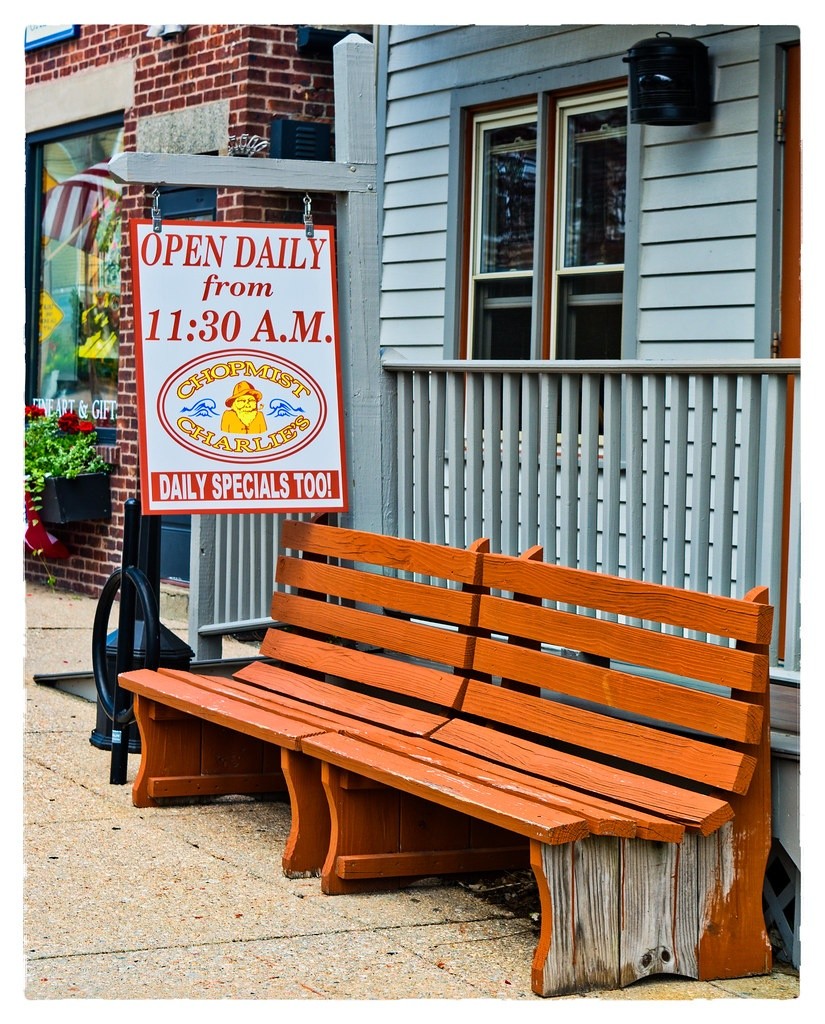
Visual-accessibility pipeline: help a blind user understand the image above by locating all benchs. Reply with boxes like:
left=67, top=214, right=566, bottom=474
left=117, top=512, right=773, bottom=998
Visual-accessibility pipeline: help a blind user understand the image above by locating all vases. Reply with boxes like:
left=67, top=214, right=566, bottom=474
left=26, top=472, right=108, bottom=525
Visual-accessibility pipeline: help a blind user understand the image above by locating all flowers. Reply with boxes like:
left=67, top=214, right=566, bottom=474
left=25, top=406, right=109, bottom=526
